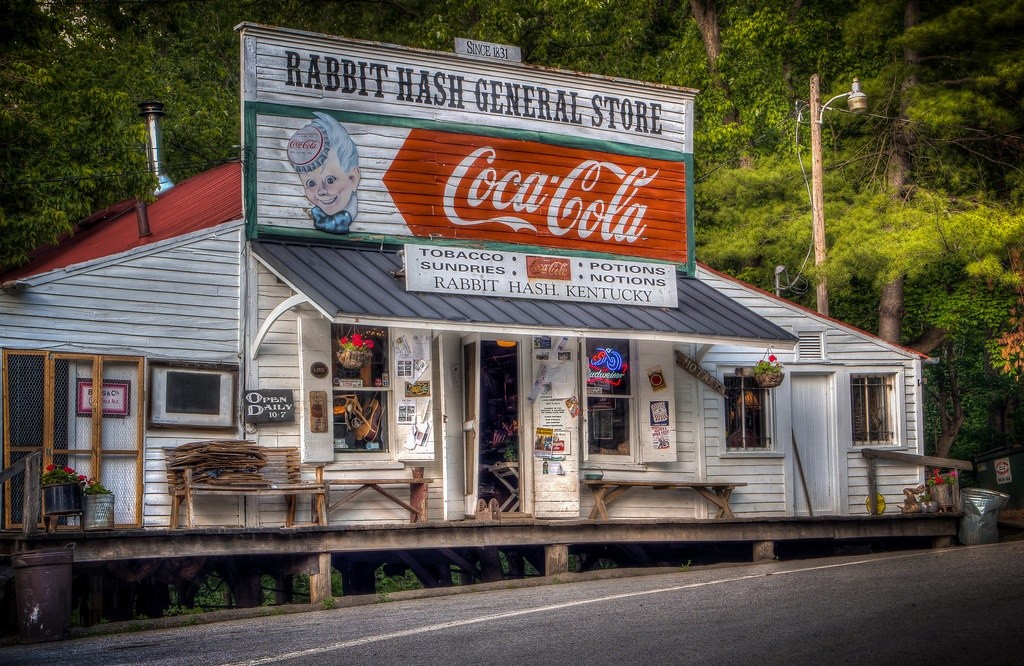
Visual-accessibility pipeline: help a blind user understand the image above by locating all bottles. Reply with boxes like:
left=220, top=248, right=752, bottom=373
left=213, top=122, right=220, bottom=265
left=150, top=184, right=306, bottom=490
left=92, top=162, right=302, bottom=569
left=543, top=461, right=548, bottom=473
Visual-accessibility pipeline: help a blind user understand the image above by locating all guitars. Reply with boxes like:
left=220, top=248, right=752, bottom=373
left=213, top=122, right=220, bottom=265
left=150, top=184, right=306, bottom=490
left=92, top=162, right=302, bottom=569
left=350, top=391, right=381, bottom=445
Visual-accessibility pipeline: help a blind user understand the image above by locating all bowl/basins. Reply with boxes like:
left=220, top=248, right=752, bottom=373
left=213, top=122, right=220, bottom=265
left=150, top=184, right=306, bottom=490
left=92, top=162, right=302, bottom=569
left=585, top=466, right=604, bottom=479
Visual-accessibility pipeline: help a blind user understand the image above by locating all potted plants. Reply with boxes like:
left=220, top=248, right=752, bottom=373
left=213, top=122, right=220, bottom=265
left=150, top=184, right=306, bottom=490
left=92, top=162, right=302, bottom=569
left=917, top=494, right=939, bottom=512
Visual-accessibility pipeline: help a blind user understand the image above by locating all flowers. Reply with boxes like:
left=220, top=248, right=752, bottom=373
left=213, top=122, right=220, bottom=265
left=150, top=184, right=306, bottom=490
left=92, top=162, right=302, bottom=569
left=753, top=355, right=785, bottom=375
left=40, top=463, right=86, bottom=486
left=83, top=477, right=113, bottom=495
left=926, top=469, right=958, bottom=496
left=337, top=333, right=375, bottom=351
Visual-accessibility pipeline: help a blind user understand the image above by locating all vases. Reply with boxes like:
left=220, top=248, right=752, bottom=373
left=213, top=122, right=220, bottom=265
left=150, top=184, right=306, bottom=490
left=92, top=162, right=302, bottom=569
left=931, top=484, right=952, bottom=508
left=335, top=352, right=373, bottom=370
left=755, top=374, right=784, bottom=389
left=40, top=484, right=86, bottom=515
left=80, top=494, right=114, bottom=530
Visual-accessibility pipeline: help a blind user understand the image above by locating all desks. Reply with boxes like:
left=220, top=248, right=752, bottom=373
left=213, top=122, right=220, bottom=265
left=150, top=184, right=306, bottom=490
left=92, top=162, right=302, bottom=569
left=488, top=462, right=522, bottom=514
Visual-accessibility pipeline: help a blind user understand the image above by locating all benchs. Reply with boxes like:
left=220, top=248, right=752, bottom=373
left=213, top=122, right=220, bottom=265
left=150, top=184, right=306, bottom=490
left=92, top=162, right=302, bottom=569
left=161, top=446, right=328, bottom=529
left=301, top=478, right=434, bottom=525
left=580, top=480, right=748, bottom=520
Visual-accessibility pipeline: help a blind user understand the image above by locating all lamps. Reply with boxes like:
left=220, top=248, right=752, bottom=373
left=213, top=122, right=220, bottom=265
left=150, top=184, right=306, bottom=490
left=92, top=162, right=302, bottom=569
left=737, top=390, right=759, bottom=409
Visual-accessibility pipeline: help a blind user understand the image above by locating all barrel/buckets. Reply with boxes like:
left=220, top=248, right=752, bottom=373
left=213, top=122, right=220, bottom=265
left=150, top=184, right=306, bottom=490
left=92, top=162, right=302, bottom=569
left=11, top=549, right=74, bottom=644
